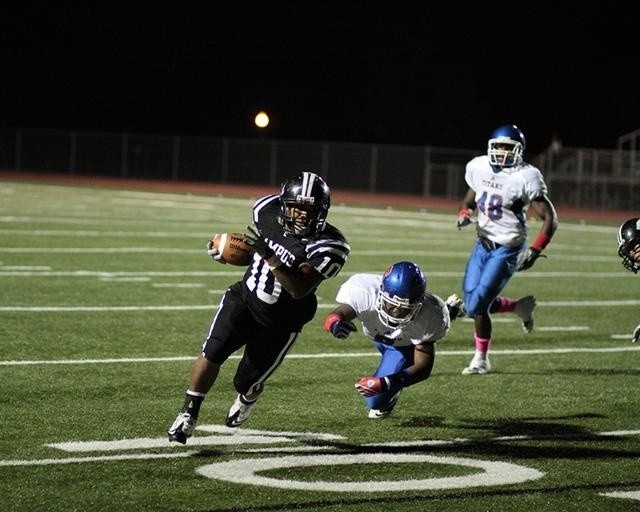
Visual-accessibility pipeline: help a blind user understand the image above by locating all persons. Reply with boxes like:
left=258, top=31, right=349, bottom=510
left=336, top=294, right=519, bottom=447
left=455, top=123, right=560, bottom=376
left=617, top=215, right=640, bottom=346
left=167, top=171, right=350, bottom=445
left=324, top=261, right=469, bottom=421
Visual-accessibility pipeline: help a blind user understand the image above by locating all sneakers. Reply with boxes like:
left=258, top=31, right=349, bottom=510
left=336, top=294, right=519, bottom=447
left=225, top=394, right=257, bottom=427
left=518, top=295, right=536, bottom=334
left=447, top=293, right=465, bottom=318
left=462, top=358, right=491, bottom=374
left=167, top=408, right=197, bottom=445
left=369, top=388, right=402, bottom=418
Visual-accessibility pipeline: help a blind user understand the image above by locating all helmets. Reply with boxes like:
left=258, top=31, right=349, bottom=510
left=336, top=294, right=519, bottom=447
left=616, top=217, right=640, bottom=274
left=375, top=261, right=427, bottom=331
left=276, top=171, right=330, bottom=241
left=487, top=124, right=526, bottom=168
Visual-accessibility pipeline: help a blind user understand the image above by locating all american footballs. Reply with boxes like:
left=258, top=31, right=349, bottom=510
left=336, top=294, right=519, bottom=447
left=211, top=234, right=249, bottom=266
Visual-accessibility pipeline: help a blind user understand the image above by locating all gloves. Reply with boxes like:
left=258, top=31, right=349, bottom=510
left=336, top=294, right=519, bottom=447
left=330, top=319, right=357, bottom=340
left=514, top=247, right=547, bottom=271
left=355, top=376, right=391, bottom=397
left=242, top=220, right=274, bottom=260
left=207, top=240, right=226, bottom=265
left=455, top=208, right=478, bottom=231
